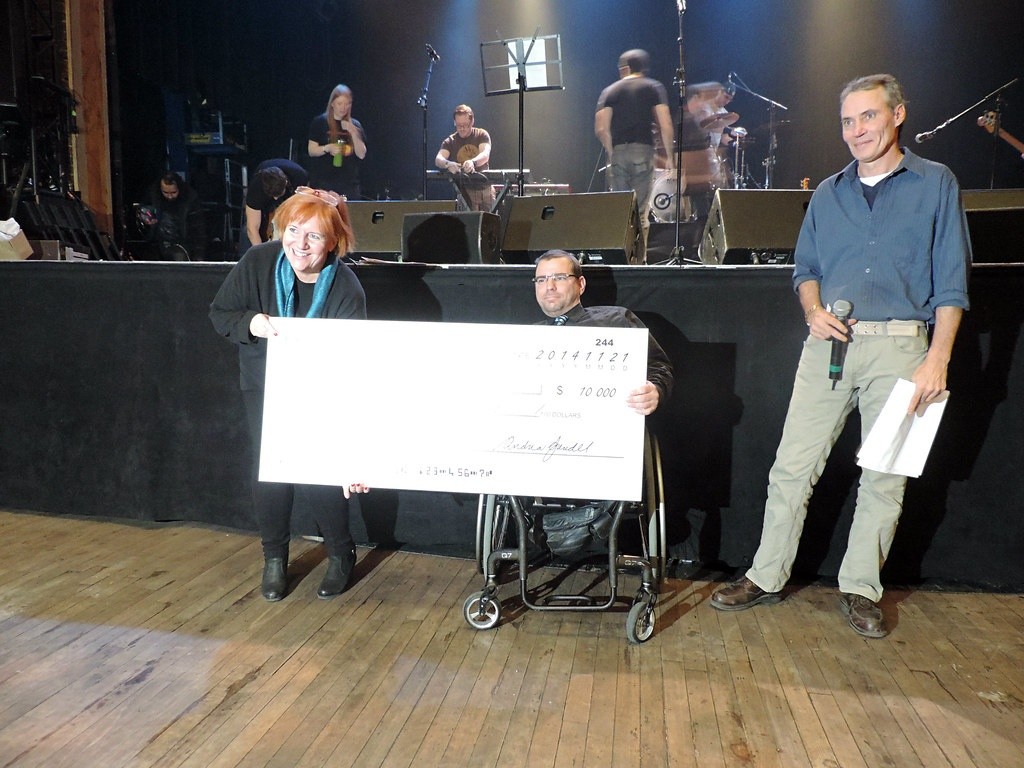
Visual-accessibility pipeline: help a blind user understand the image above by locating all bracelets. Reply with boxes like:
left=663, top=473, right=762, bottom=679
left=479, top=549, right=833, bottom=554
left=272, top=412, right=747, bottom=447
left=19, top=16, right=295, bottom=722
left=472, top=160, right=476, bottom=168
left=445, top=162, right=450, bottom=169
left=804, top=304, right=823, bottom=323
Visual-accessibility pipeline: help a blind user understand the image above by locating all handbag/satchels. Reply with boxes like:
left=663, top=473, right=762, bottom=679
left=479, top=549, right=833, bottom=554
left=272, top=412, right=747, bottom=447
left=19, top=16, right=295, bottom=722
left=649, top=167, right=690, bottom=225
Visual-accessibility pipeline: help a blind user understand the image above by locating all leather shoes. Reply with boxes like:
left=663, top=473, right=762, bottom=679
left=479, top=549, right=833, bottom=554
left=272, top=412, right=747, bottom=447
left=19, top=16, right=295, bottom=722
left=316, top=550, right=358, bottom=599
left=841, top=592, right=889, bottom=638
left=260, top=557, right=289, bottom=602
left=709, top=576, right=790, bottom=611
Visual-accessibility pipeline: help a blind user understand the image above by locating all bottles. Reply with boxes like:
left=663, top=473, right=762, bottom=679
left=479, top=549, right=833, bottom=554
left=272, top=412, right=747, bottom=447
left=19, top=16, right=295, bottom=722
left=333, top=140, right=343, bottom=167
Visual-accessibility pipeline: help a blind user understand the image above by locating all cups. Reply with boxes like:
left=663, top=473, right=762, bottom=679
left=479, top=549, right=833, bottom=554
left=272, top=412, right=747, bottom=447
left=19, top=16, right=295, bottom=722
left=800, top=176, right=810, bottom=190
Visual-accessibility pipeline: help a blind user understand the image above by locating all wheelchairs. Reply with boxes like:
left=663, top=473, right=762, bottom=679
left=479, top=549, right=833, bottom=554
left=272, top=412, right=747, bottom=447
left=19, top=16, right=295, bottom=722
left=463, top=423, right=666, bottom=645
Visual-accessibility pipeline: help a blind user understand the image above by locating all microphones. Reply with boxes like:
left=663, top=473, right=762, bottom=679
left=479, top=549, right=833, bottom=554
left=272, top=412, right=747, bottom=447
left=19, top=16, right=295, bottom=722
left=915, top=132, right=934, bottom=143
left=677, top=0, right=687, bottom=13
left=426, top=44, right=440, bottom=63
left=829, top=300, right=851, bottom=390
left=658, top=197, right=666, bottom=204
left=728, top=73, right=732, bottom=90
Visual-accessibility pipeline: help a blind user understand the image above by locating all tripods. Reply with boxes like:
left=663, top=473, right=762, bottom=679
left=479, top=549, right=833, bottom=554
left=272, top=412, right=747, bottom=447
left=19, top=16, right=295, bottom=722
left=654, top=12, right=703, bottom=267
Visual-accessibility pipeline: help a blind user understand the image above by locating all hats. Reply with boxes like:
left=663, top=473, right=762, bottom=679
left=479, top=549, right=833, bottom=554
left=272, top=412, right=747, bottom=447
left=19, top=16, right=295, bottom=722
left=722, top=81, right=737, bottom=97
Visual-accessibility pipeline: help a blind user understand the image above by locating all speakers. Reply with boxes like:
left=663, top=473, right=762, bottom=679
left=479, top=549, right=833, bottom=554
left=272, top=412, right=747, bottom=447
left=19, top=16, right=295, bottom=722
left=342, top=197, right=466, bottom=262
left=960, top=189, right=1024, bottom=264
left=503, top=188, right=646, bottom=266
left=401, top=211, right=503, bottom=265
left=698, top=189, right=816, bottom=266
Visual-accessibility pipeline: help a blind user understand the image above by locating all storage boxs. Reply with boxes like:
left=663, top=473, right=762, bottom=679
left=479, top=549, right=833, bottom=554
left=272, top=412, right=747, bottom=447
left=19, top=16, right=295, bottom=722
left=0, top=229, right=90, bottom=261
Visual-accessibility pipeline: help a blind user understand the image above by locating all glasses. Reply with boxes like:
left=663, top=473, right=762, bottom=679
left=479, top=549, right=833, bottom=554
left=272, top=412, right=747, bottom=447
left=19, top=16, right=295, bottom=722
left=453, top=119, right=472, bottom=129
left=531, top=272, right=576, bottom=285
left=294, top=185, right=339, bottom=207
left=723, top=94, right=735, bottom=104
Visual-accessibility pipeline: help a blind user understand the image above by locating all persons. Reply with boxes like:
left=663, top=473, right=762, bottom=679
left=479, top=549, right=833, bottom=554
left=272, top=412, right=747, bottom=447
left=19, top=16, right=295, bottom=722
left=309, top=85, right=367, bottom=199
left=435, top=105, right=496, bottom=212
left=151, top=172, right=207, bottom=261
left=596, top=50, right=747, bottom=263
left=240, top=159, right=309, bottom=255
left=709, top=75, right=973, bottom=639
left=535, top=250, right=676, bottom=415
left=210, top=190, right=369, bottom=602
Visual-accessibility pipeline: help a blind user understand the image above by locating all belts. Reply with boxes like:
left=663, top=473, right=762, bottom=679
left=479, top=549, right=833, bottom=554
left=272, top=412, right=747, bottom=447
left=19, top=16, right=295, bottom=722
left=850, top=319, right=925, bottom=338
